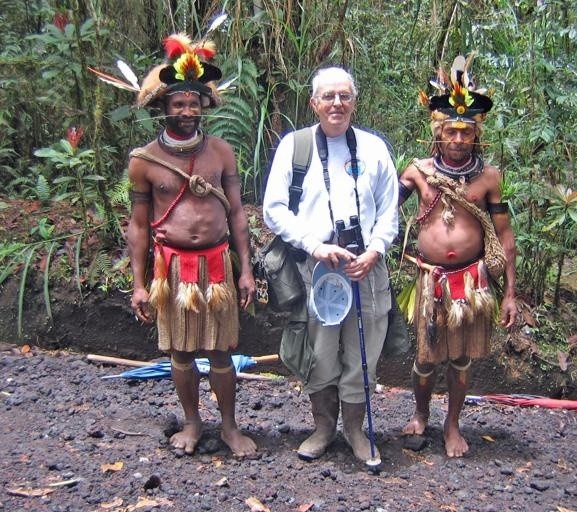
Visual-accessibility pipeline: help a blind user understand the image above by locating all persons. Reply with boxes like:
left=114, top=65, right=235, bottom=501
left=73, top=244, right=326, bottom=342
left=395, top=84, right=518, bottom=458
left=259, top=66, right=402, bottom=468
left=124, top=55, right=260, bottom=459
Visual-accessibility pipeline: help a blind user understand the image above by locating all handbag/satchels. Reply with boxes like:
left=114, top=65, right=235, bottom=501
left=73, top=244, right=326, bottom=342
left=484, top=222, right=508, bottom=280
left=252, top=234, right=308, bottom=313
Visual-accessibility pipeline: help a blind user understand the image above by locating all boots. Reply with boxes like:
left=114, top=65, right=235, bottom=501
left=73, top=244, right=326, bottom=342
left=341, top=400, right=380, bottom=463
left=297, top=385, right=339, bottom=459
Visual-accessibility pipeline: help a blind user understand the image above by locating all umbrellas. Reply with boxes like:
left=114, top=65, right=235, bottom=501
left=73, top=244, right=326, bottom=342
left=98, top=353, right=281, bottom=380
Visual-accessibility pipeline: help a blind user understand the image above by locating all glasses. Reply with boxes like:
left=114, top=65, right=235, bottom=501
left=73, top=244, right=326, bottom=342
left=315, top=90, right=354, bottom=104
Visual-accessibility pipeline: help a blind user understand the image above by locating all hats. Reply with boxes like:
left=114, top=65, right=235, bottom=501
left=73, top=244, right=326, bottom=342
left=428, top=91, right=493, bottom=124
left=136, top=61, right=222, bottom=111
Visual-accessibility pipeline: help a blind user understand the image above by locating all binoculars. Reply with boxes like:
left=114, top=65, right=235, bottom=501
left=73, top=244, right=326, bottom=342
left=335, top=215, right=366, bottom=261
